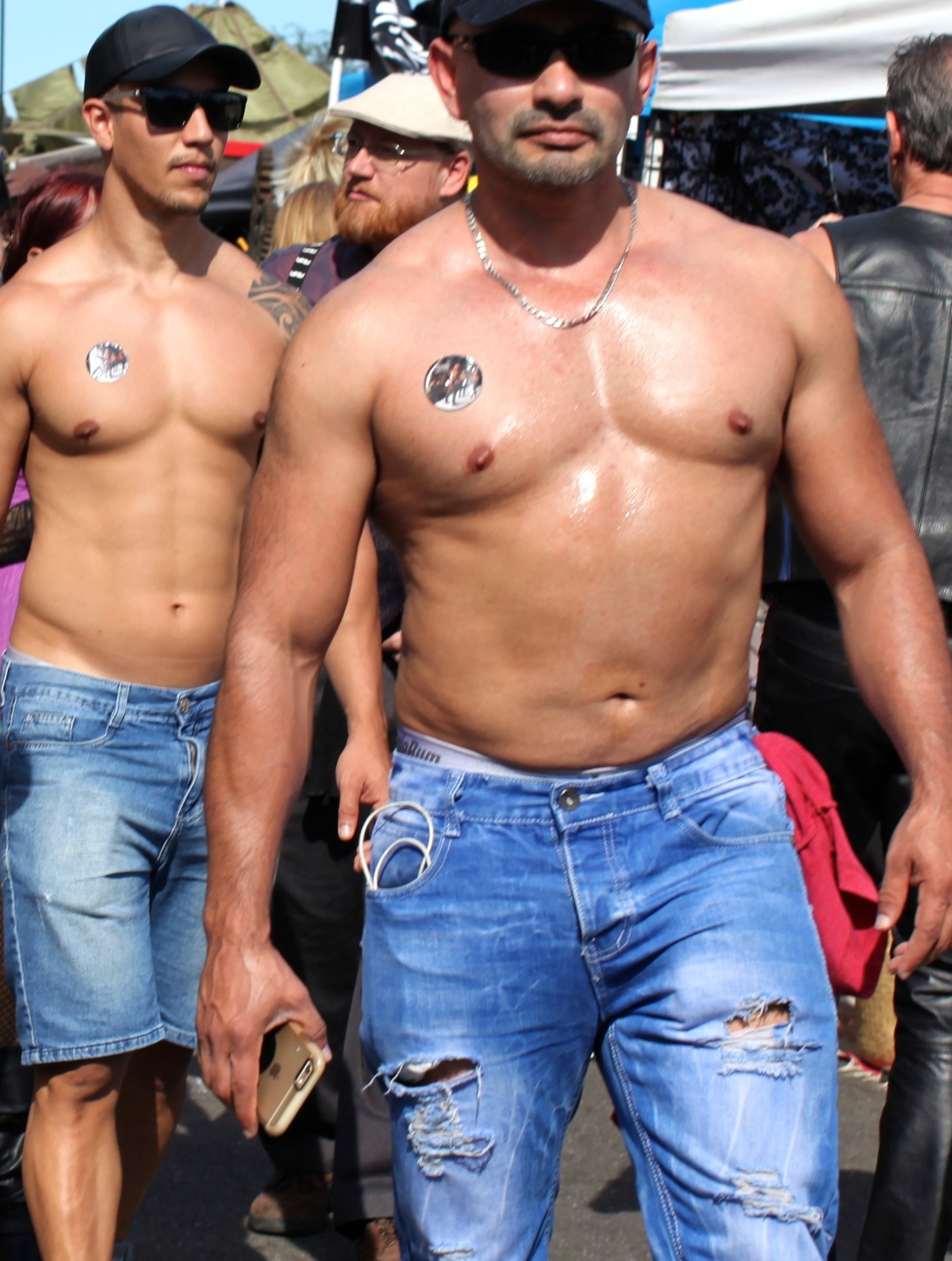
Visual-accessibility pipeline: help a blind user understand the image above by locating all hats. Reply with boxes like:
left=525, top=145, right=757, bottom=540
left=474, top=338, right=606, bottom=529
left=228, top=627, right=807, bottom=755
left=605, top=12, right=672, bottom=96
left=454, top=0, right=656, bottom=36
left=329, top=72, right=475, bottom=143
left=82, top=4, right=261, bottom=101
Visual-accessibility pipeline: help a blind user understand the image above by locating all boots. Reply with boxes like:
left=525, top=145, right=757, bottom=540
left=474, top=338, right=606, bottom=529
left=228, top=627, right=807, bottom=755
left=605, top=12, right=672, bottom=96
left=856, top=930, right=896, bottom=1070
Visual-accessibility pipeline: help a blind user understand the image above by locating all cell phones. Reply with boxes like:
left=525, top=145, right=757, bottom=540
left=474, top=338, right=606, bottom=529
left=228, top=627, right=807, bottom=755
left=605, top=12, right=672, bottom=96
left=252, top=1020, right=327, bottom=1137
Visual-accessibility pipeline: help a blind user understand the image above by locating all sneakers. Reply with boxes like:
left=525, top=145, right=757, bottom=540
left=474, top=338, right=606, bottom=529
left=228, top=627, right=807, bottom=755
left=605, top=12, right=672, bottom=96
left=248, top=1167, right=336, bottom=1233
left=362, top=1217, right=401, bottom=1261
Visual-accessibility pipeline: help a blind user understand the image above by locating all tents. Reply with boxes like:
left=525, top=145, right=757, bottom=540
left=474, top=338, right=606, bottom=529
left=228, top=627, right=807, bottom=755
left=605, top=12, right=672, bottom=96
left=638, top=0, right=952, bottom=192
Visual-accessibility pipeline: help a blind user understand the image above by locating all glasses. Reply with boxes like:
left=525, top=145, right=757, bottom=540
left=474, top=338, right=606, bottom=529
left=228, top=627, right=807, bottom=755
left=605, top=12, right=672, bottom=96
left=331, top=131, right=442, bottom=163
left=102, top=90, right=246, bottom=132
left=444, top=7, right=643, bottom=78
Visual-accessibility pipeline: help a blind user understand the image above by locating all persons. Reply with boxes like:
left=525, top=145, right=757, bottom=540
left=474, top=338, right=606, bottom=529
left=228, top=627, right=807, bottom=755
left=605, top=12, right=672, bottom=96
left=748, top=33, right=952, bottom=1261
left=195, top=0, right=952, bottom=1261
left=1, top=5, right=478, bottom=1261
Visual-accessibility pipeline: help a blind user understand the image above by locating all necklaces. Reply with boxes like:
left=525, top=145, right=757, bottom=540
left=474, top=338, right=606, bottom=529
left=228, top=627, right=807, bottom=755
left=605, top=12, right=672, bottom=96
left=458, top=174, right=640, bottom=330
